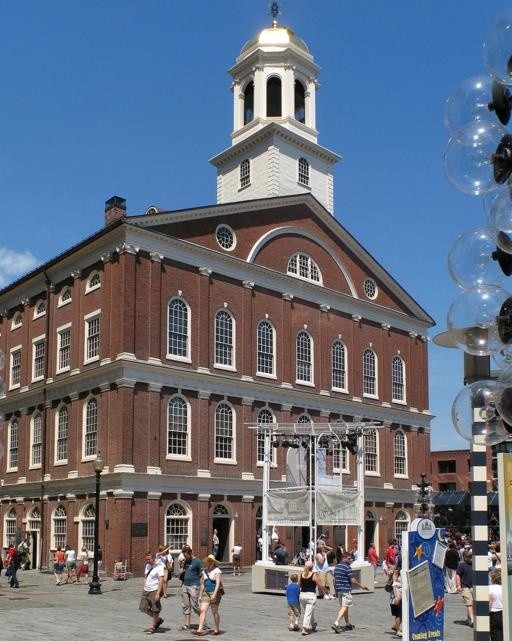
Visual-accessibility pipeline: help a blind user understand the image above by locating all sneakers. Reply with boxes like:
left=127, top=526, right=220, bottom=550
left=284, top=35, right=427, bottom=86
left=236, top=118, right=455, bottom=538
left=323, top=593, right=336, bottom=600
left=289, top=622, right=318, bottom=635
left=331, top=623, right=355, bottom=632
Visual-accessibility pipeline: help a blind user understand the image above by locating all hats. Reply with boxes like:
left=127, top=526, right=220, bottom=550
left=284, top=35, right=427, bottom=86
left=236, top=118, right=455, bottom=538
left=202, top=554, right=222, bottom=566
left=305, top=561, right=314, bottom=568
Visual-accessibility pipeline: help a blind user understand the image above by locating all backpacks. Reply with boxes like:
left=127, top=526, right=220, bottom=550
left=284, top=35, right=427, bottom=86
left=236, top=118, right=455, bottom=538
left=11, top=546, right=25, bottom=562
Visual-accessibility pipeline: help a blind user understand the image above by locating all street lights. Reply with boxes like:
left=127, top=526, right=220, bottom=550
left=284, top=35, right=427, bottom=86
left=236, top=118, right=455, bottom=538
left=88, top=447, right=108, bottom=594
left=440, top=20, right=511, bottom=447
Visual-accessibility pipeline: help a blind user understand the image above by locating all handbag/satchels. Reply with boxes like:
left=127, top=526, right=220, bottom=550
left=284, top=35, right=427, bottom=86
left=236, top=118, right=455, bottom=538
left=219, top=582, right=224, bottom=595
left=53, top=558, right=65, bottom=566
left=5, top=568, right=16, bottom=575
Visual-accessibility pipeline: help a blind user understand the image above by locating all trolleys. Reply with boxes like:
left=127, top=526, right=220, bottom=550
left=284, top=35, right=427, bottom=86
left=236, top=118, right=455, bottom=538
left=113, top=557, right=129, bottom=580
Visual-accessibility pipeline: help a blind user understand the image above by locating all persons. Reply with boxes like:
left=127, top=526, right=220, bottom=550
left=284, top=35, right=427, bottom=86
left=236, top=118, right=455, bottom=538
left=52, top=545, right=89, bottom=585
left=97, top=544, right=102, bottom=577
left=139, top=544, right=222, bottom=635
left=434, top=512, right=503, bottom=641
left=5, top=539, right=31, bottom=588
left=257, top=523, right=402, bottom=637
left=213, top=529, right=220, bottom=557
left=231, top=543, right=242, bottom=576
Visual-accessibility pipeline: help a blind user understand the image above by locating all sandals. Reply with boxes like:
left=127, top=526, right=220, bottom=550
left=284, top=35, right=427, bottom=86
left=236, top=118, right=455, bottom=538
left=178, top=625, right=219, bottom=636
left=145, top=618, right=164, bottom=634
